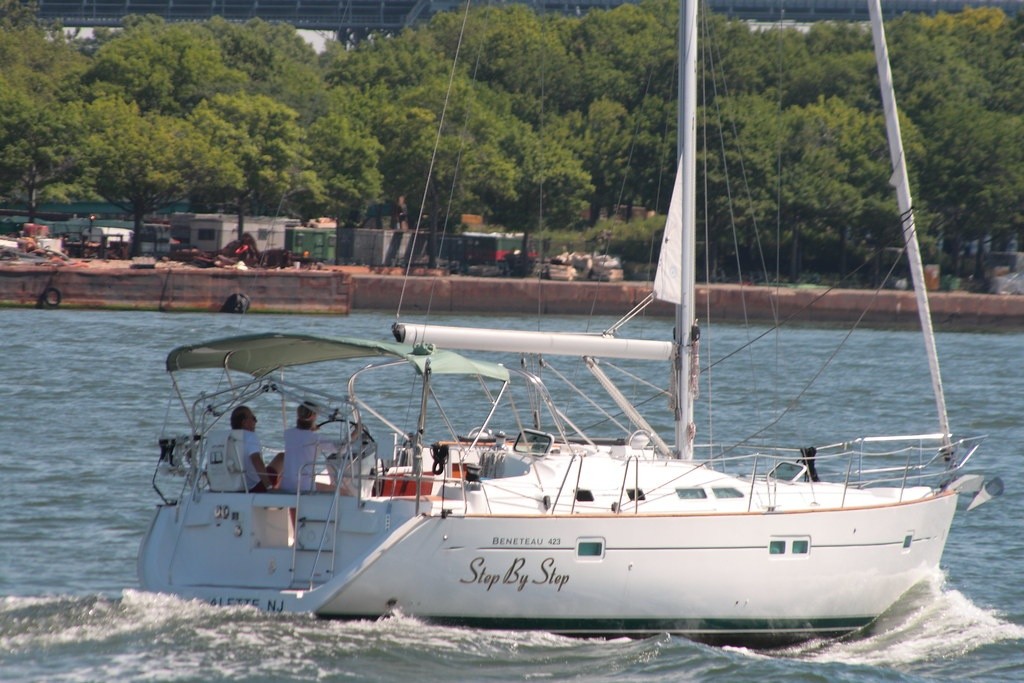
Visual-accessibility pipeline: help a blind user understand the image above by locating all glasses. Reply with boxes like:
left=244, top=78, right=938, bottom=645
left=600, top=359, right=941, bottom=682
left=251, top=417, right=257, bottom=422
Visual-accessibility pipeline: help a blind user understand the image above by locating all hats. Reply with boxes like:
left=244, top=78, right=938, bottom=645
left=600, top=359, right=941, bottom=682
left=297, top=401, right=316, bottom=422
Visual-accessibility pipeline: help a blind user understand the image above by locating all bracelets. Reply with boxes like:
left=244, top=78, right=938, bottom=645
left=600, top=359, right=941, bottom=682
left=265, top=485, right=273, bottom=490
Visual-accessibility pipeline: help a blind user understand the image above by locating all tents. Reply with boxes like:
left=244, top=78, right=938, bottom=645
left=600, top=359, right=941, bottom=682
left=165, top=332, right=510, bottom=470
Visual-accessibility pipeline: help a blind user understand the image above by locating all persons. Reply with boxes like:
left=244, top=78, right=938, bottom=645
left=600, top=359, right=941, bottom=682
left=594, top=238, right=606, bottom=256
left=358, top=432, right=372, bottom=449
left=551, top=246, right=569, bottom=265
left=403, top=432, right=415, bottom=448
left=280, top=401, right=362, bottom=494
left=225, top=405, right=285, bottom=493
